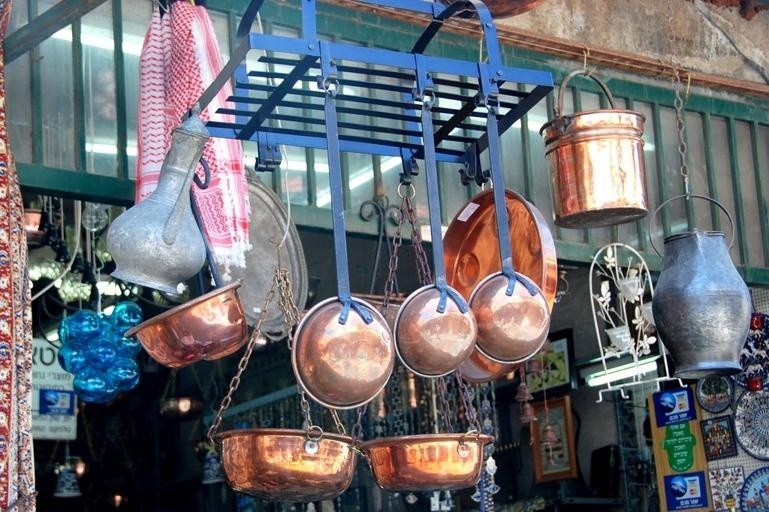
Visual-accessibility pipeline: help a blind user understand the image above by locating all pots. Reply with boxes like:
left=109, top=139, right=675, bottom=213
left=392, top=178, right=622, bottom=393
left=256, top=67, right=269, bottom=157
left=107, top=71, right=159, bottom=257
left=393, top=91, right=480, bottom=379
left=469, top=88, right=550, bottom=366
left=442, top=177, right=558, bottom=386
left=358, top=426, right=498, bottom=494
left=291, top=91, right=396, bottom=411
left=211, top=427, right=365, bottom=498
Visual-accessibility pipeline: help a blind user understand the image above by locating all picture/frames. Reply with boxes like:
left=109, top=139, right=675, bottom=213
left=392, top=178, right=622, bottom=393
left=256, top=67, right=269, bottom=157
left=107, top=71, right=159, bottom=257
left=529, top=395, right=579, bottom=484
left=526, top=328, right=578, bottom=402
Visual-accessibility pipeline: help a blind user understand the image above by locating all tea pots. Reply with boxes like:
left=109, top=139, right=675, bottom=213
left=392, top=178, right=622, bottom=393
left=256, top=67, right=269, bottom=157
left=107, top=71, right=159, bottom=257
left=105, top=102, right=211, bottom=295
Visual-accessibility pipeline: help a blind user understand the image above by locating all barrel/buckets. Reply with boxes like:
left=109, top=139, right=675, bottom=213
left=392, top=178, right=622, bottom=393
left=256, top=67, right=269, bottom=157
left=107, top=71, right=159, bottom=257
left=540, top=71, right=647, bottom=228
